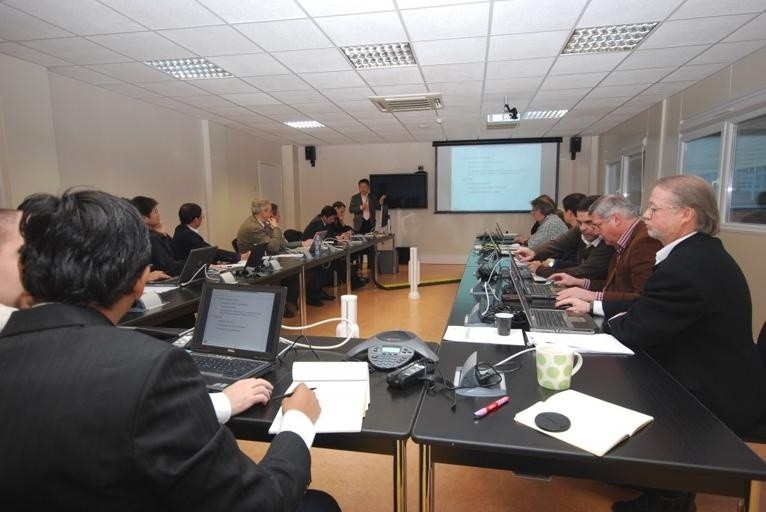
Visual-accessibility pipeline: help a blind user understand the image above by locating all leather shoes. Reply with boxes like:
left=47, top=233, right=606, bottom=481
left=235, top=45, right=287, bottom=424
left=316, top=292, right=337, bottom=300
left=351, top=269, right=370, bottom=291
left=283, top=304, right=294, bottom=318
left=306, top=295, right=325, bottom=307
left=610, top=492, right=693, bottom=512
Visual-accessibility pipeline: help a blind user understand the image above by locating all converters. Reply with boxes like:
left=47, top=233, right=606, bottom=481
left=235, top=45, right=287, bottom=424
left=386, top=359, right=435, bottom=386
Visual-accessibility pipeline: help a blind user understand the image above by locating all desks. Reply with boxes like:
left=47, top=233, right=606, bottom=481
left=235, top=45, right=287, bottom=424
left=144, top=327, right=440, bottom=512
left=412, top=231, right=766, bottom=512
left=118, top=232, right=396, bottom=335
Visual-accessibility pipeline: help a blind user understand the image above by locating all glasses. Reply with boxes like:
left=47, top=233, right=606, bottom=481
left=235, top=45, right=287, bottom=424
left=153, top=208, right=160, bottom=215
left=590, top=217, right=610, bottom=233
left=202, top=215, right=206, bottom=219
left=123, top=262, right=155, bottom=293
left=640, top=204, right=683, bottom=219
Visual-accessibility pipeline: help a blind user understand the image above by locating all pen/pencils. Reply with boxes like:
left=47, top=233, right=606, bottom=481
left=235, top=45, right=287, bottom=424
left=270, top=386, right=317, bottom=401
left=473, top=394, right=512, bottom=418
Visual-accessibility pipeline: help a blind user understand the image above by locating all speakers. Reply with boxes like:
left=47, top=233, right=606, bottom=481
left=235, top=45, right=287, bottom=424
left=571, top=135, right=583, bottom=153
left=305, top=145, right=316, bottom=159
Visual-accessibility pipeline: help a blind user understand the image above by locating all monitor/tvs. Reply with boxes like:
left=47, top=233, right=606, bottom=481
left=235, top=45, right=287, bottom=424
left=371, top=173, right=427, bottom=209
left=381, top=205, right=388, bottom=227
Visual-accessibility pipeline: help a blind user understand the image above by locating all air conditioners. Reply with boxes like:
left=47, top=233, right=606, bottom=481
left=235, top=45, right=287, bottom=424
left=369, top=93, right=444, bottom=113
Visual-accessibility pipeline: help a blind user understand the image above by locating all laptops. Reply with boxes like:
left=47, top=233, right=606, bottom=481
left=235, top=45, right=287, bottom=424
left=496, top=222, right=519, bottom=239
left=509, top=248, right=567, bottom=299
left=292, top=230, right=328, bottom=254
left=185, top=282, right=288, bottom=392
left=510, top=262, right=600, bottom=334
left=226, top=241, right=271, bottom=274
left=488, top=232, right=524, bottom=255
left=146, top=245, right=219, bottom=285
left=496, top=228, right=516, bottom=243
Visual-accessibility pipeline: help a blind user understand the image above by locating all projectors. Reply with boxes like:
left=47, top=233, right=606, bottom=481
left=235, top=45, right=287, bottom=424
left=486, top=95, right=521, bottom=130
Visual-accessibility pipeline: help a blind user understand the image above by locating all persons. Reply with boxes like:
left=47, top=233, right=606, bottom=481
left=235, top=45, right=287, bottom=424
left=546, top=193, right=664, bottom=304
left=333, top=201, right=370, bottom=291
left=236, top=197, right=294, bottom=319
left=0, top=183, right=342, bottom=512
left=554, top=174, right=765, bottom=512
left=0, top=208, right=272, bottom=422
left=349, top=178, right=387, bottom=271
left=525, top=195, right=615, bottom=281
left=132, top=196, right=185, bottom=277
left=269, top=203, right=312, bottom=309
left=146, top=270, right=171, bottom=283
left=513, top=194, right=572, bottom=247
left=562, top=192, right=586, bottom=226
left=528, top=198, right=568, bottom=250
left=173, top=203, right=251, bottom=265
left=301, top=205, right=349, bottom=307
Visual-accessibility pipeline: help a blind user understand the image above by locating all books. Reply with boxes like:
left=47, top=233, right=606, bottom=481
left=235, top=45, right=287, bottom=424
left=268, top=360, right=371, bottom=435
left=513, top=389, right=654, bottom=457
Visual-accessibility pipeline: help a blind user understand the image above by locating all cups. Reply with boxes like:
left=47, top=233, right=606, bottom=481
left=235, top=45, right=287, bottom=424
left=536, top=344, right=583, bottom=390
left=495, top=313, right=514, bottom=336
